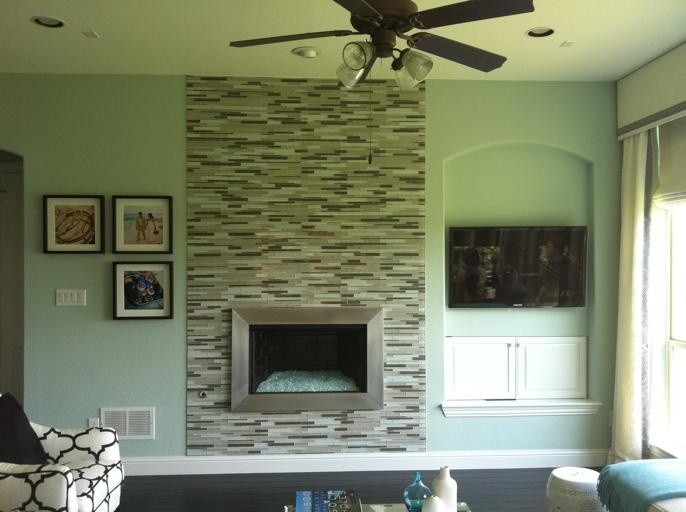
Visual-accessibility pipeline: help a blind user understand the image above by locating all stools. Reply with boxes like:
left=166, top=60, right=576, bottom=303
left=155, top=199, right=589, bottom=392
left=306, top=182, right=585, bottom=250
left=547, top=455, right=686, bottom=512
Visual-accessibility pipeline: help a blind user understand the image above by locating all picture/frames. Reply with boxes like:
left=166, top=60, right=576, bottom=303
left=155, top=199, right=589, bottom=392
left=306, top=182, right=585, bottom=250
left=40, top=193, right=175, bottom=321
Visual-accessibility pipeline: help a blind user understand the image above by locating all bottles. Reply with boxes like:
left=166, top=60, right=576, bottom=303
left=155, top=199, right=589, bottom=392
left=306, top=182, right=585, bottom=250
left=403, top=466, right=457, bottom=512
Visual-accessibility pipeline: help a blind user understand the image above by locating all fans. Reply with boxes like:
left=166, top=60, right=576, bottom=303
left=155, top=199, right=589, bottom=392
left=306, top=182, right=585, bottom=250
left=228, top=0, right=537, bottom=74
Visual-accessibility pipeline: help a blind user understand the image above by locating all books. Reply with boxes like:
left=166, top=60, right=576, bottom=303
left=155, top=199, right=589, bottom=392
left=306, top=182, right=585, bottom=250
left=294, top=488, right=364, bottom=512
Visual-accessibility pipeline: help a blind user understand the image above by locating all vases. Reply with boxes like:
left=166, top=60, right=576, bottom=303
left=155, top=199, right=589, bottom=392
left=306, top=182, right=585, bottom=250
left=403, top=464, right=459, bottom=512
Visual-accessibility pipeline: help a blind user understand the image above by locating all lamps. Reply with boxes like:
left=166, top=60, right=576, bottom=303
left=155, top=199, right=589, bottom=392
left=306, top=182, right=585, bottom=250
left=335, top=29, right=436, bottom=95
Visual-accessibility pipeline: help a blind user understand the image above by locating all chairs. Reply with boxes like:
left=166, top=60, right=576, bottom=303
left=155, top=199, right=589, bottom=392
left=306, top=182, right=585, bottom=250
left=0, top=420, right=127, bottom=511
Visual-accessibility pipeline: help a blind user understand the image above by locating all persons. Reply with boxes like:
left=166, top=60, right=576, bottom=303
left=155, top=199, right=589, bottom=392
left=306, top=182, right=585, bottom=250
left=134, top=211, right=146, bottom=242
left=145, top=213, right=159, bottom=242
left=452, top=248, right=488, bottom=305
left=534, top=231, right=582, bottom=306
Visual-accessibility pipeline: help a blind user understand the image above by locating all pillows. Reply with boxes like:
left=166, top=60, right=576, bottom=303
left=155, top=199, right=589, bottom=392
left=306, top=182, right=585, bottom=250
left=0, top=389, right=48, bottom=464
